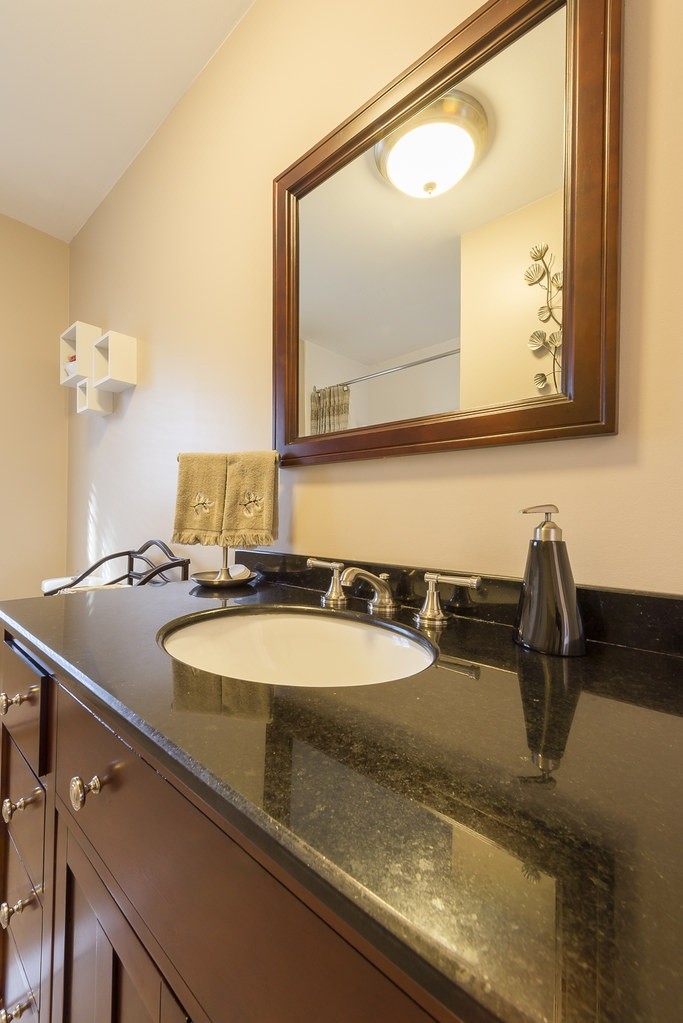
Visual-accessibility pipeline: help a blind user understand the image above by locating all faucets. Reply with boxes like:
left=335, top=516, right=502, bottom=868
left=342, top=567, right=402, bottom=613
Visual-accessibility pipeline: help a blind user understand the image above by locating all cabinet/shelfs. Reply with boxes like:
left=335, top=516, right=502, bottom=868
left=0, top=618, right=503, bottom=1023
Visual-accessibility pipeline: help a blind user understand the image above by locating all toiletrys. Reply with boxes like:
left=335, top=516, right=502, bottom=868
left=510, top=660, right=582, bottom=793
left=515, top=504, right=587, bottom=657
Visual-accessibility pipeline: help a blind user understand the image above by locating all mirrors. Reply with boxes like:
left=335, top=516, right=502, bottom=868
left=271, top=0, right=626, bottom=470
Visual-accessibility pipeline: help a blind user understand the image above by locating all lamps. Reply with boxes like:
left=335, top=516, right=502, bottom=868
left=369, top=82, right=495, bottom=201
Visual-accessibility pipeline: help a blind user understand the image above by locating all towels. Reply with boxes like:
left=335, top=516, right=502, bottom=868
left=172, top=450, right=279, bottom=550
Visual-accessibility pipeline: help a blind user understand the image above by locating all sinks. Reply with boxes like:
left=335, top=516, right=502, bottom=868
left=155, top=605, right=439, bottom=688
